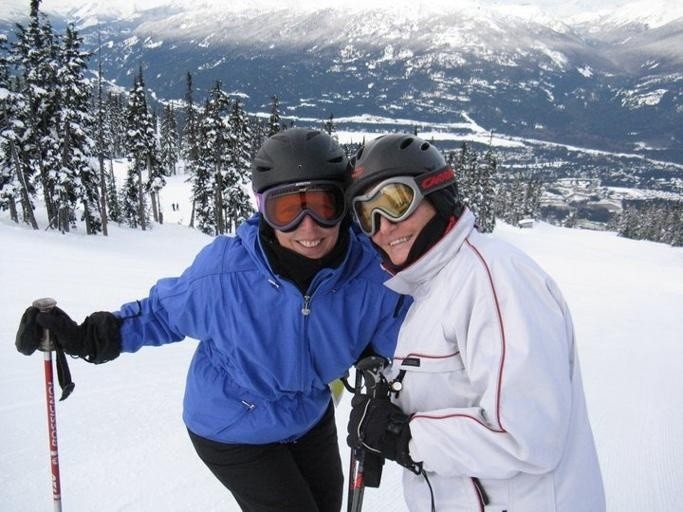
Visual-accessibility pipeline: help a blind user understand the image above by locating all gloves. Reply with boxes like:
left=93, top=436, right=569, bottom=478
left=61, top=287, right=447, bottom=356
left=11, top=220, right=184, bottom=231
left=15, top=305, right=91, bottom=356
left=347, top=393, right=423, bottom=475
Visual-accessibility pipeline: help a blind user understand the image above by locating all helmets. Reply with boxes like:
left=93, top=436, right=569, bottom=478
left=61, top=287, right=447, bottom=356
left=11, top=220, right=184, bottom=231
left=252, top=127, right=348, bottom=194
left=345, top=133, right=446, bottom=214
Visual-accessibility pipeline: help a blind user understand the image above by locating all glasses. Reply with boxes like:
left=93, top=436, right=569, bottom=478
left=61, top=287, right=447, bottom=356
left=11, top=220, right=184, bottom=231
left=252, top=179, right=346, bottom=232
left=350, top=162, right=455, bottom=237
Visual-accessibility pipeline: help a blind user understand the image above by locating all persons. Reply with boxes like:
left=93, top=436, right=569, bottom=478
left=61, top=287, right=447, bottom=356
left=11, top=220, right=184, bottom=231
left=345, top=134, right=605, bottom=511
left=14, top=123, right=413, bottom=512
